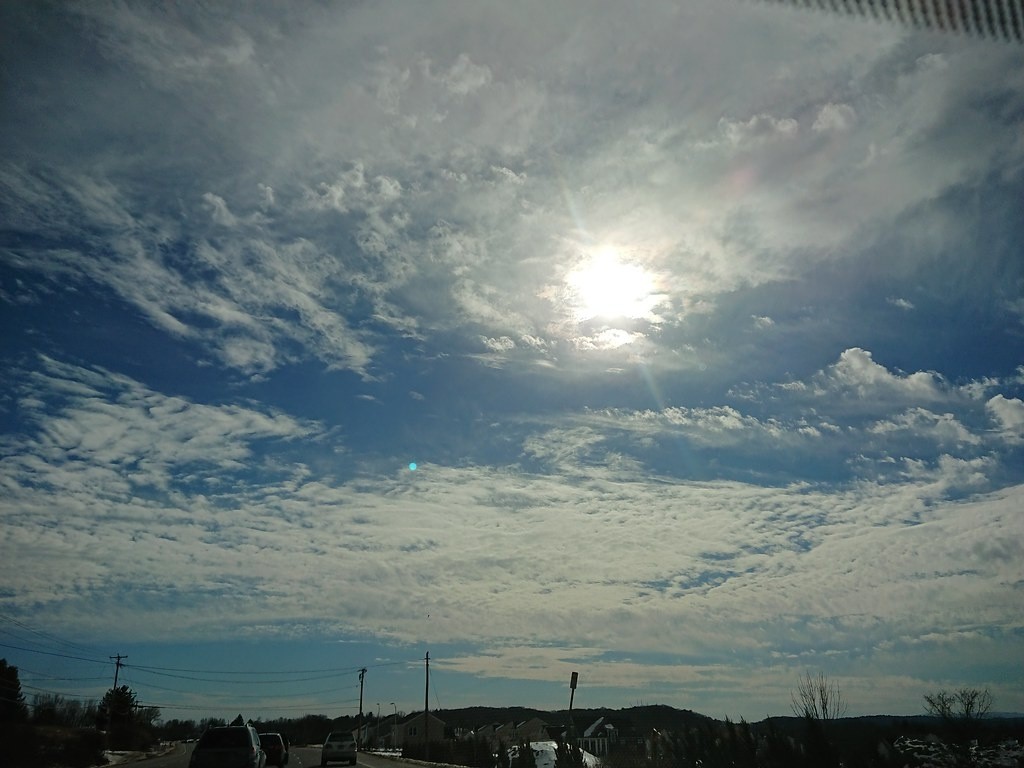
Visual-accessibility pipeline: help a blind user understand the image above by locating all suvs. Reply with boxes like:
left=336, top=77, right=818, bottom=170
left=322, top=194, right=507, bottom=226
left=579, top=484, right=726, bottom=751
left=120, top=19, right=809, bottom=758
left=188, top=723, right=266, bottom=768
left=321, top=731, right=357, bottom=767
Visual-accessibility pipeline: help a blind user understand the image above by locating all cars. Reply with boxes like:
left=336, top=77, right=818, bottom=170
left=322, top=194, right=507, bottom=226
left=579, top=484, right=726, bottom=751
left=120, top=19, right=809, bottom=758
left=178, top=739, right=200, bottom=744
left=259, top=732, right=290, bottom=768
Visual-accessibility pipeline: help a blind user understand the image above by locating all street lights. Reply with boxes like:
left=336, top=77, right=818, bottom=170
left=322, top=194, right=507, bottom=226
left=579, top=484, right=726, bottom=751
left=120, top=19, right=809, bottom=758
left=376, top=703, right=380, bottom=742
left=389, top=703, right=396, bottom=753
